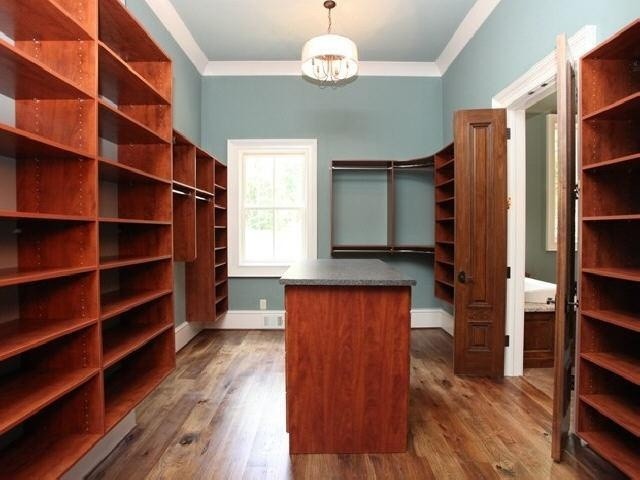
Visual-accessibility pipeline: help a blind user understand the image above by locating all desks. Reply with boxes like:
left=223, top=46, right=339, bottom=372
left=277, top=258, right=417, bottom=454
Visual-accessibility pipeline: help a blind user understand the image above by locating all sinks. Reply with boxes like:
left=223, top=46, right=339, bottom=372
left=524, top=276, right=557, bottom=305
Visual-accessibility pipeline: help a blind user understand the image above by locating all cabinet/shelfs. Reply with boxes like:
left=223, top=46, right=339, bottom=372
left=98, top=0, right=178, bottom=437
left=185, top=158, right=228, bottom=321
left=435, top=141, right=454, bottom=305
left=0, top=0, right=103, bottom=479
left=173, top=126, right=214, bottom=203
left=573, top=17, right=640, bottom=479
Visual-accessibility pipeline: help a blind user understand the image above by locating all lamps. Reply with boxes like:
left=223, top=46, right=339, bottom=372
left=300, top=0, right=360, bottom=88
